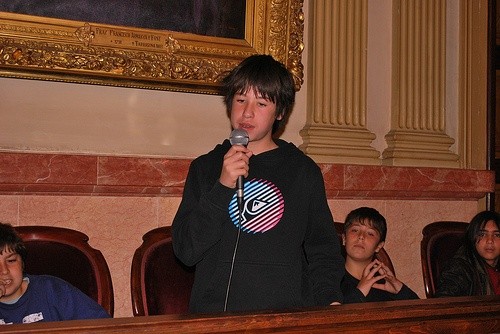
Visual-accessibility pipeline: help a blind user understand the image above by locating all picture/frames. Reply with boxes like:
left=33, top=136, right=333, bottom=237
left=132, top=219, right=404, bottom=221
left=0, top=0, right=307, bottom=97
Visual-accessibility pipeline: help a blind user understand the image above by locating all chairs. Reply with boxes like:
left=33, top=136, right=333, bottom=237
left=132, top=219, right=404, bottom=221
left=128, top=225, right=193, bottom=316
left=418, top=219, right=471, bottom=299
left=6, top=223, right=117, bottom=320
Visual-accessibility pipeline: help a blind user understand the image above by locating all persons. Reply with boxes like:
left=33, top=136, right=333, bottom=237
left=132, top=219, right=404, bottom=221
left=0, top=221, right=110, bottom=325
left=340, top=207, right=421, bottom=303
left=171, top=54, right=346, bottom=310
left=442, top=210, right=500, bottom=297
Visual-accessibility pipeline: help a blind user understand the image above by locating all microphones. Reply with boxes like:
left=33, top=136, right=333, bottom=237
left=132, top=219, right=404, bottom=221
left=229, top=127, right=249, bottom=208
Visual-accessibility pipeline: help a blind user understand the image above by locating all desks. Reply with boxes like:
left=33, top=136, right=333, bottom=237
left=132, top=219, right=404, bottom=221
left=0, top=293, right=500, bottom=334
